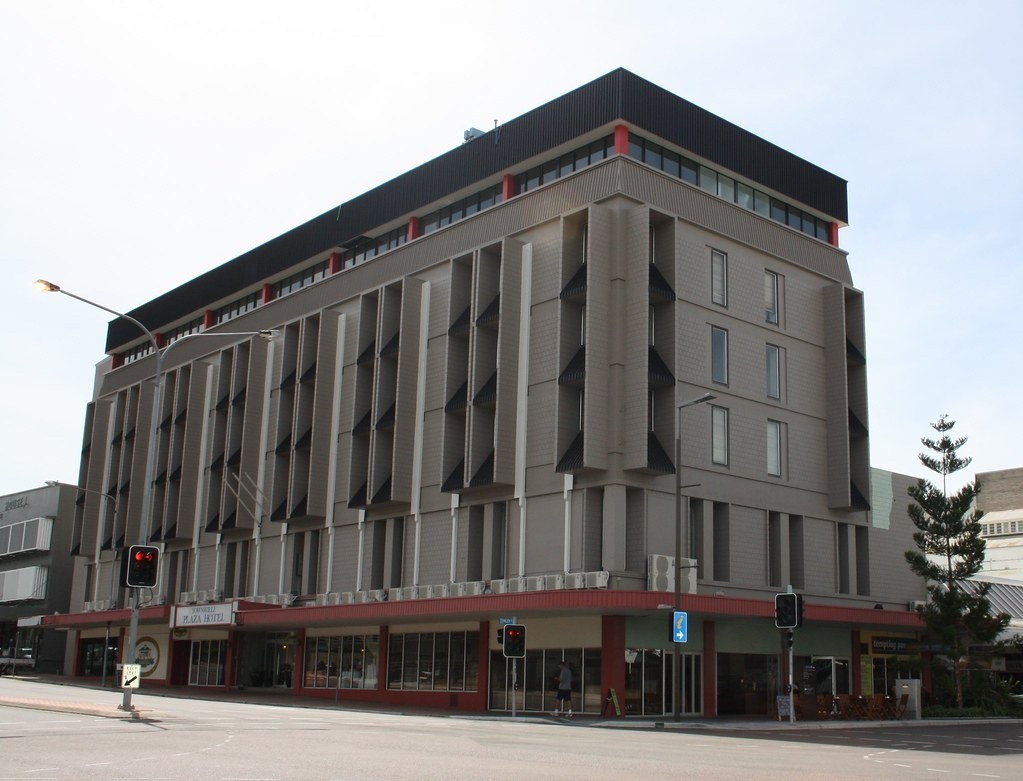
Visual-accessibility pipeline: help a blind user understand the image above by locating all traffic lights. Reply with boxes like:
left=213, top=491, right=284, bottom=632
left=120, top=545, right=160, bottom=588
left=774, top=592, right=797, bottom=629
left=502, top=624, right=526, bottom=658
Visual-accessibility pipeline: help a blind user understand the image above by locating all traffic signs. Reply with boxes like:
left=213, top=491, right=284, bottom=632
left=121, top=664, right=142, bottom=689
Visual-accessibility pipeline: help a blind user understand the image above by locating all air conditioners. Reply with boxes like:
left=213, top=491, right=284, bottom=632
left=648, top=554, right=698, bottom=594
left=909, top=600, right=926, bottom=612
left=129, top=595, right=164, bottom=608
left=84, top=600, right=115, bottom=611
left=225, top=594, right=293, bottom=606
left=389, top=581, right=482, bottom=600
left=181, top=590, right=218, bottom=601
left=316, top=590, right=384, bottom=606
left=490, top=571, right=607, bottom=594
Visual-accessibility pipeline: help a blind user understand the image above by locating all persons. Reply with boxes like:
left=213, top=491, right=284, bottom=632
left=549, top=661, right=572, bottom=719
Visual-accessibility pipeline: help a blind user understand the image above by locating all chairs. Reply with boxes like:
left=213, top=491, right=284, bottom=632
left=793, top=694, right=908, bottom=720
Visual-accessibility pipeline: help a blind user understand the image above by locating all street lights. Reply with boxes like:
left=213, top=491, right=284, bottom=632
left=669, top=392, right=717, bottom=726
left=32, top=279, right=283, bottom=709
left=45, top=480, right=118, bottom=688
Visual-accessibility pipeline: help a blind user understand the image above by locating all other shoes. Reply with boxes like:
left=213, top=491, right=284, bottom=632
left=549, top=712, right=559, bottom=717
left=565, top=712, right=572, bottom=717
